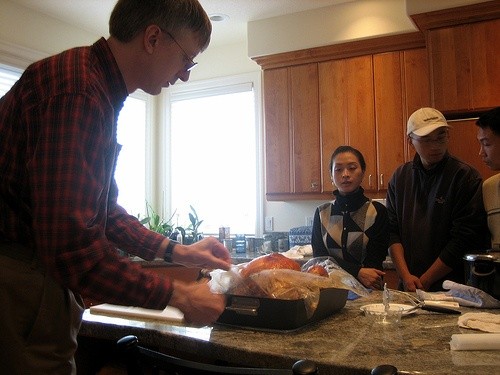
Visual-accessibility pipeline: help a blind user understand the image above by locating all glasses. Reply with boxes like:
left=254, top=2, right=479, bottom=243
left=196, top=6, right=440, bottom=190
left=410, top=132, right=448, bottom=148
left=160, top=27, right=199, bottom=73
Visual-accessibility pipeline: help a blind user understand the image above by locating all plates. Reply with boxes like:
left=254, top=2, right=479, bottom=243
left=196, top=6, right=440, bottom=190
left=359, top=304, right=418, bottom=316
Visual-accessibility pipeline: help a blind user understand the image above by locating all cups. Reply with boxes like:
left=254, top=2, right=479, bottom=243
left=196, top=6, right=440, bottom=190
left=223, top=239, right=233, bottom=252
left=278, top=239, right=289, bottom=252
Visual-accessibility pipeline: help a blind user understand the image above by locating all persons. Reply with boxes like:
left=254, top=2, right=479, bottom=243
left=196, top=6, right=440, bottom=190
left=311, top=146, right=389, bottom=290
left=0, top=0, right=231, bottom=375
left=476, top=106, right=500, bottom=299
left=386, top=107, right=487, bottom=292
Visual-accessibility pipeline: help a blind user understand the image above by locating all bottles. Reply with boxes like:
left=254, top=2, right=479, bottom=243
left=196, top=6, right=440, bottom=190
left=235, top=233, right=246, bottom=253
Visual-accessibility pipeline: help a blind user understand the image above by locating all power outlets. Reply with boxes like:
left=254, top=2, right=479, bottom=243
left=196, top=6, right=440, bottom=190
left=305, top=215, right=315, bottom=227
left=265, top=216, right=273, bottom=230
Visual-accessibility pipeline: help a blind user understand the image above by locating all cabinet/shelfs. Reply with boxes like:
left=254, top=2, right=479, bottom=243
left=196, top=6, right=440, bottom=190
left=261, top=17, right=500, bottom=203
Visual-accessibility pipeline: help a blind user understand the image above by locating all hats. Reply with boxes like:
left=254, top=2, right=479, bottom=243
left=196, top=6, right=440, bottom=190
left=406, top=107, right=453, bottom=136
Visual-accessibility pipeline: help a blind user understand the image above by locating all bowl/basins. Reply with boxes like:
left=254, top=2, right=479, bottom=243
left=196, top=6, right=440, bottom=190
left=363, top=304, right=404, bottom=326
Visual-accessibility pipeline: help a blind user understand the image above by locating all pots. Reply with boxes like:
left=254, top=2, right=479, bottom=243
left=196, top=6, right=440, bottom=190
left=215, top=286, right=348, bottom=330
left=462, top=249, right=500, bottom=301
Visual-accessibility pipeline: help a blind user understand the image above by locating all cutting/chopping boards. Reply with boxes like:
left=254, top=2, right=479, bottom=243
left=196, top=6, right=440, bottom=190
left=89, top=302, right=188, bottom=323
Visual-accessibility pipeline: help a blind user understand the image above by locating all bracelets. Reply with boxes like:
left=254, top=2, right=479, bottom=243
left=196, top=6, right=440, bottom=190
left=164, top=241, right=179, bottom=262
